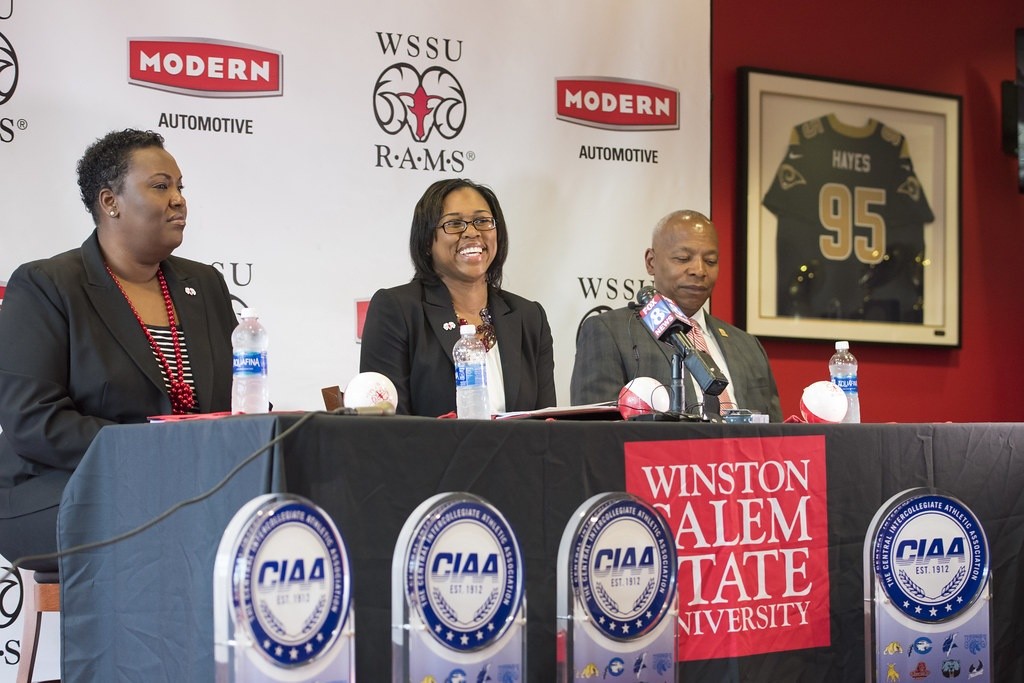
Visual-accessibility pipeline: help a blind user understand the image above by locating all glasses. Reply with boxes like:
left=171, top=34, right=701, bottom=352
left=437, top=215, right=497, bottom=234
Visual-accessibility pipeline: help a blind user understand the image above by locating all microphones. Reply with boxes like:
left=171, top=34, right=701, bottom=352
left=637, top=286, right=729, bottom=396
left=628, top=301, right=671, bottom=366
left=334, top=401, right=396, bottom=417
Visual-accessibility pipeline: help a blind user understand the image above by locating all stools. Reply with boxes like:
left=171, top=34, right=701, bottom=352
left=16, top=571, right=60, bottom=683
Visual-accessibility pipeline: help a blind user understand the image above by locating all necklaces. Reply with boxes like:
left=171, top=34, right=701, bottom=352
left=104, top=264, right=196, bottom=416
left=455, top=307, right=496, bottom=351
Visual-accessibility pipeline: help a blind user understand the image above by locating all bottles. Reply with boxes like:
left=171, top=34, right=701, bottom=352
left=230, top=309, right=270, bottom=415
left=829, top=340, right=860, bottom=424
left=452, top=324, right=491, bottom=421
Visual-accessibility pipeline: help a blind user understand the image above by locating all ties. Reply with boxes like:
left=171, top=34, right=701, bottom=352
left=686, top=319, right=734, bottom=416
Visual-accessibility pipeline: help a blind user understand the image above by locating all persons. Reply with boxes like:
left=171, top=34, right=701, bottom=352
left=357, top=178, right=559, bottom=414
left=0, top=127, right=236, bottom=573
left=571, top=211, right=783, bottom=426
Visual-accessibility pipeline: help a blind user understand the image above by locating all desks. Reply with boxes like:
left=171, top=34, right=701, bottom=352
left=58, top=417, right=1024, bottom=683
